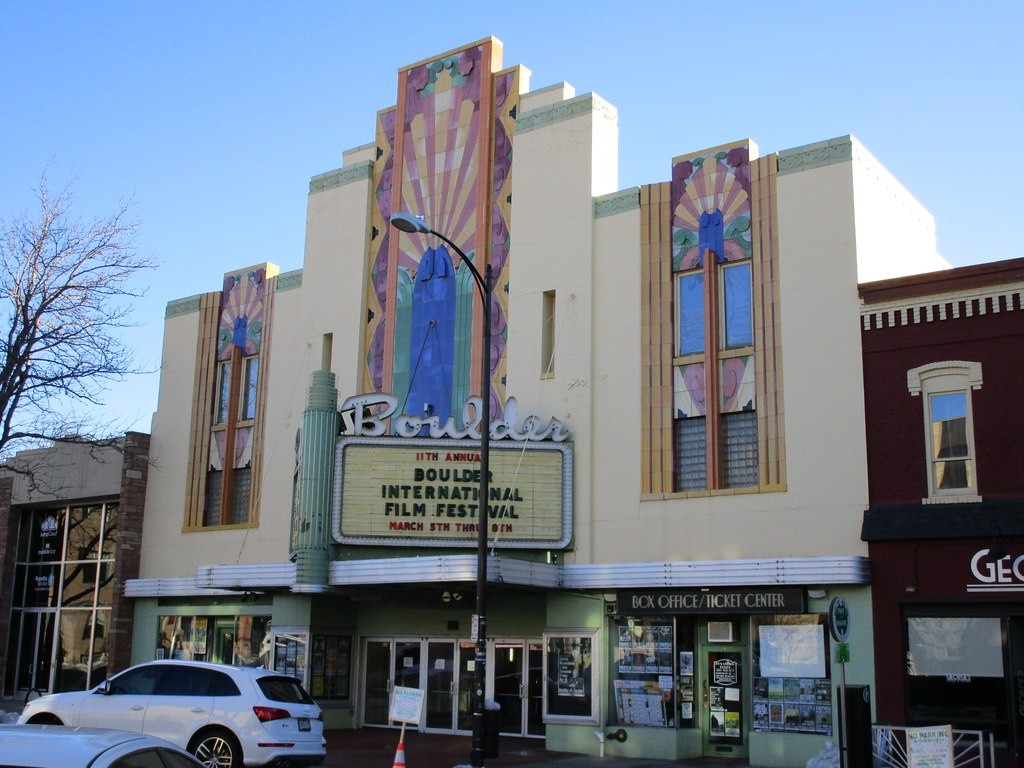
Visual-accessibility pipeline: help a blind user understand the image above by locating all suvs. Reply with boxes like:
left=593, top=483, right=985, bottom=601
left=16, top=659, right=328, bottom=768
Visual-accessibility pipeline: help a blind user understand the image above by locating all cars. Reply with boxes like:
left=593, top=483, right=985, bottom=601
left=0, top=723, right=209, bottom=768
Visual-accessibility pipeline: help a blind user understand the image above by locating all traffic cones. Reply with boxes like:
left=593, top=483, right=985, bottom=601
left=392, top=741, right=405, bottom=768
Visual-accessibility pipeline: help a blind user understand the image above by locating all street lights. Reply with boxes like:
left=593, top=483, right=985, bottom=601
left=391, top=212, right=493, bottom=768
